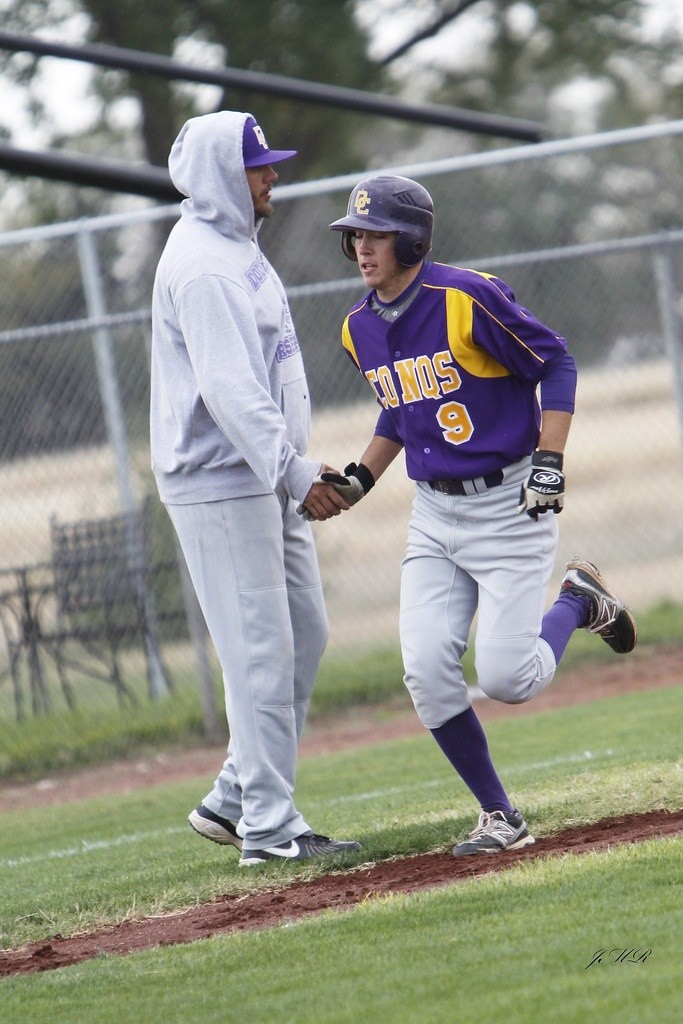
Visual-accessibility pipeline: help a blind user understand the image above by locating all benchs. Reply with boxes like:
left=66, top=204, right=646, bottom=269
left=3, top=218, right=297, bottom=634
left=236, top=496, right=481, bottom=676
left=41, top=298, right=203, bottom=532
left=0, top=498, right=181, bottom=726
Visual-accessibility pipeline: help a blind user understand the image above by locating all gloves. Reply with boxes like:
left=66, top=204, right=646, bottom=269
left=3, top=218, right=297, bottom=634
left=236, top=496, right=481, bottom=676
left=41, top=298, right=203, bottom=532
left=296, top=460, right=374, bottom=524
left=516, top=450, right=566, bottom=523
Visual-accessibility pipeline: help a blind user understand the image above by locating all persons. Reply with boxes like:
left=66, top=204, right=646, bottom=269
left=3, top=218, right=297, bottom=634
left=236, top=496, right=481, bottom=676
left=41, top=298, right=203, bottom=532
left=292, top=175, right=638, bottom=859
left=145, top=109, right=363, bottom=872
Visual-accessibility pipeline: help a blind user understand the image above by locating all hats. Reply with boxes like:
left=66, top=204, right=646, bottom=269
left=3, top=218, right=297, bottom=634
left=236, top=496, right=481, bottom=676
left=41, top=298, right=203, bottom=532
left=328, top=178, right=436, bottom=271
left=237, top=117, right=298, bottom=170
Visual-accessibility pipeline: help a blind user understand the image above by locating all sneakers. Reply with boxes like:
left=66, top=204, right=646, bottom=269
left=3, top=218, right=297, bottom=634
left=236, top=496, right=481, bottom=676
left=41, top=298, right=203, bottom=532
left=189, top=802, right=246, bottom=855
left=236, top=833, right=363, bottom=872
left=561, top=561, right=638, bottom=655
left=450, top=807, right=538, bottom=861
